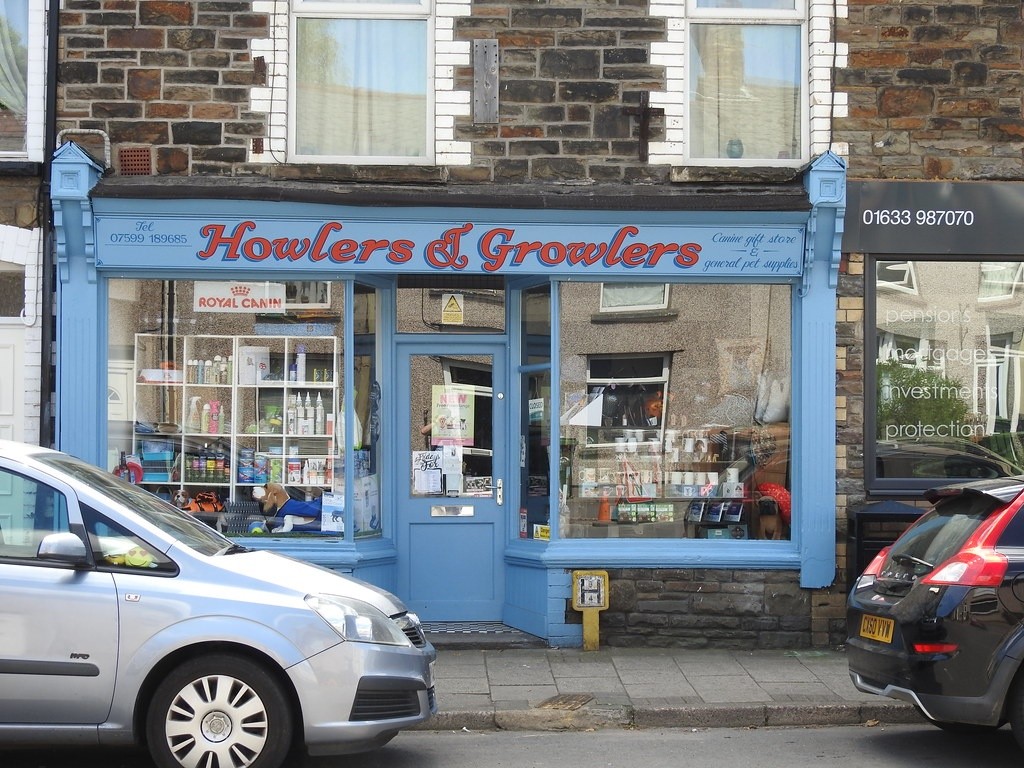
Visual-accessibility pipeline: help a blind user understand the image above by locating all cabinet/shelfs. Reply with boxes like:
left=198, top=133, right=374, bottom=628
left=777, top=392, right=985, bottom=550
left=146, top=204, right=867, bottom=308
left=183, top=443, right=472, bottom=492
left=131, top=332, right=341, bottom=497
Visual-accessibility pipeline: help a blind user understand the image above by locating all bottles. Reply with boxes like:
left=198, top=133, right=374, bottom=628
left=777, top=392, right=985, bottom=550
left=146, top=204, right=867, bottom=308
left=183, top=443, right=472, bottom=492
left=185, top=443, right=229, bottom=479
left=227, top=356, right=232, bottom=384
left=198, top=360, right=204, bottom=384
left=221, top=357, right=227, bottom=384
left=213, top=355, right=221, bottom=384
left=194, top=360, right=198, bottom=383
left=297, top=391, right=302, bottom=407
left=305, top=392, right=311, bottom=407
left=316, top=392, right=323, bottom=406
left=304, top=464, right=310, bottom=484
left=219, top=406, right=224, bottom=434
left=187, top=359, right=193, bottom=383
left=204, top=360, right=211, bottom=383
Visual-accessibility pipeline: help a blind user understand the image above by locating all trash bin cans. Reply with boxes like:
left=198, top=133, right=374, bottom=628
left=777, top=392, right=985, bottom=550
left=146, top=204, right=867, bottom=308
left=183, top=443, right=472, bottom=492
left=846, top=499, right=930, bottom=599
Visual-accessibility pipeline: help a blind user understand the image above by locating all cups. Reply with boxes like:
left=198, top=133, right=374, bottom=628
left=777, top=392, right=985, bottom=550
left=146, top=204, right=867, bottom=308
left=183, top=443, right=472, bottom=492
left=590, top=488, right=599, bottom=496
left=582, top=487, right=589, bottom=496
left=579, top=468, right=595, bottom=481
left=695, top=473, right=706, bottom=485
left=641, top=470, right=652, bottom=483
left=616, top=486, right=628, bottom=495
left=617, top=430, right=710, bottom=452
left=727, top=468, right=739, bottom=483
left=683, top=472, right=694, bottom=484
left=610, top=472, right=625, bottom=485
left=603, top=486, right=613, bottom=496
left=596, top=468, right=612, bottom=483
left=670, top=473, right=682, bottom=484
left=706, top=473, right=718, bottom=485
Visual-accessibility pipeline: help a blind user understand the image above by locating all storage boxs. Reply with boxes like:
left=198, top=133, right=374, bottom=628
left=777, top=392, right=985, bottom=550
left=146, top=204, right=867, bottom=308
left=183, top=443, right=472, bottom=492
left=321, top=490, right=363, bottom=537
left=288, top=458, right=302, bottom=484
left=338, top=473, right=379, bottom=533
left=141, top=460, right=175, bottom=482
left=140, top=438, right=176, bottom=461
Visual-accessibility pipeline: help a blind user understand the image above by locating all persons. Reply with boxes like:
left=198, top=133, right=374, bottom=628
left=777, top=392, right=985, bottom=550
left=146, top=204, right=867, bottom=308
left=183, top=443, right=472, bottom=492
left=421, top=408, right=463, bottom=497
left=635, top=385, right=664, bottom=426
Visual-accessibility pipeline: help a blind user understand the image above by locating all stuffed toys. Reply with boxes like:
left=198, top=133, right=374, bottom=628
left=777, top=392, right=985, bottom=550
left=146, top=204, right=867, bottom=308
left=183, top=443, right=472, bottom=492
left=174, top=490, right=226, bottom=512
left=253, top=484, right=322, bottom=533
left=754, top=491, right=790, bottom=540
left=125, top=546, right=152, bottom=568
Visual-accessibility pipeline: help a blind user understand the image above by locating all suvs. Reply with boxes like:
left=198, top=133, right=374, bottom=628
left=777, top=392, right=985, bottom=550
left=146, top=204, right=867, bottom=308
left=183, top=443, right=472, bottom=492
left=844, top=475, right=1024, bottom=752
left=683, top=433, right=1024, bottom=583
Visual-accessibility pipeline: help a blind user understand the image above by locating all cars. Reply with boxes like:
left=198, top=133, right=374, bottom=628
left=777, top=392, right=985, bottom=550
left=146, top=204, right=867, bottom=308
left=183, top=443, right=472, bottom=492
left=106, top=420, right=339, bottom=516
left=0, top=439, right=434, bottom=768
